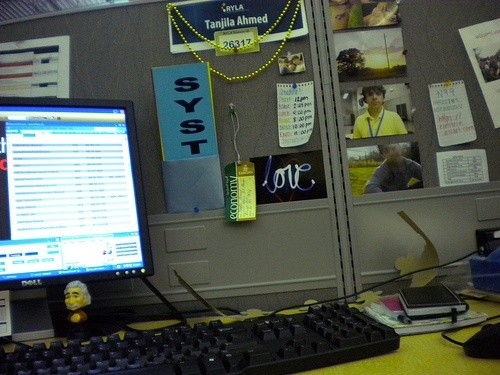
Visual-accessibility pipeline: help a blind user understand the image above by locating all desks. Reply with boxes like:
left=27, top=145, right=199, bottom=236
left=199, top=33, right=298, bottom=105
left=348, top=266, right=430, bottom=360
left=0, top=291, right=500, bottom=375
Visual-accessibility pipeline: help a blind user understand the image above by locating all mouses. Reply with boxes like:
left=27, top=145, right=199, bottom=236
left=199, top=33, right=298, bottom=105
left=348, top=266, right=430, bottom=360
left=463, top=322, right=500, bottom=359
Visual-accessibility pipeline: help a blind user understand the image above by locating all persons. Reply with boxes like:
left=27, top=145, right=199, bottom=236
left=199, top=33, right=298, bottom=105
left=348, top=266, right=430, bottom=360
left=352, top=85, right=408, bottom=139
left=365, top=143, right=424, bottom=194
left=279, top=54, right=304, bottom=73
left=62, top=280, right=104, bottom=342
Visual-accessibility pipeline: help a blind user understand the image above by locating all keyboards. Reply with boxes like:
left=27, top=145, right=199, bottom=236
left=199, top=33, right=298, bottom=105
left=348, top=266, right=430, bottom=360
left=0, top=301, right=401, bottom=375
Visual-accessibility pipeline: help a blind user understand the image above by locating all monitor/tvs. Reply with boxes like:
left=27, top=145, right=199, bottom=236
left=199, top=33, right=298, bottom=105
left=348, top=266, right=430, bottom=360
left=0, top=96, right=155, bottom=291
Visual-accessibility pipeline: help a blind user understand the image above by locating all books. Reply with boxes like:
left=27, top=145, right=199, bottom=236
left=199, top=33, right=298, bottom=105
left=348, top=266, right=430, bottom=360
left=365, top=281, right=488, bottom=336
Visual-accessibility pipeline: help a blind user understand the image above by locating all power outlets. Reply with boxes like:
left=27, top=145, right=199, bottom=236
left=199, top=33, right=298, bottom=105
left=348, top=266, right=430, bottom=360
left=476, top=227, right=500, bottom=257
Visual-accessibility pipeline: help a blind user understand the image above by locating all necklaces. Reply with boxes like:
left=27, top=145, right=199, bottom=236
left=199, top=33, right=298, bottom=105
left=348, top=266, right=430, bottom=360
left=367, top=108, right=384, bottom=138
